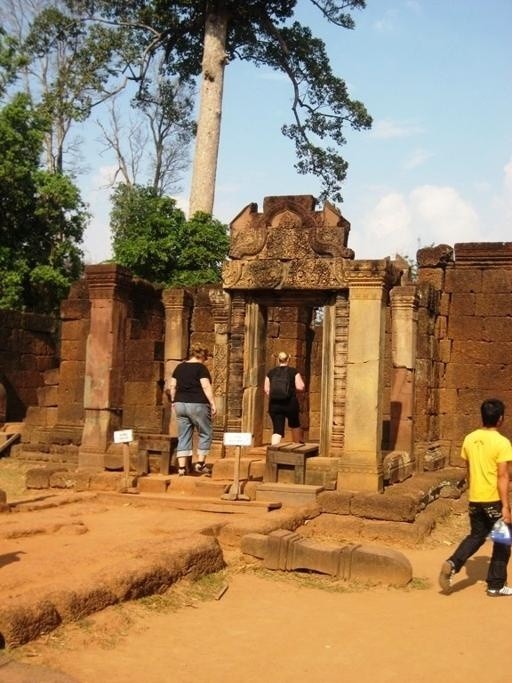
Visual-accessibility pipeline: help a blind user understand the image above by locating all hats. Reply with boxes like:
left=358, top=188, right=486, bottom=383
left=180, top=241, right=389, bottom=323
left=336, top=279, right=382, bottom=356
left=490, top=517, right=512, bottom=557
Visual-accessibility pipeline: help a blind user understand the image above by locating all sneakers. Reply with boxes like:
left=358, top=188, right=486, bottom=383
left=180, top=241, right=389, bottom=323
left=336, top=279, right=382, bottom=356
left=487, top=585, right=512, bottom=596
left=178, top=468, right=190, bottom=475
left=194, top=463, right=211, bottom=474
left=439, top=560, right=456, bottom=594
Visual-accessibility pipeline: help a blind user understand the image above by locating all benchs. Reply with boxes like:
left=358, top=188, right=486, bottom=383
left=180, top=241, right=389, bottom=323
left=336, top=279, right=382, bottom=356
left=266, top=442, right=320, bottom=485
left=136, top=433, right=193, bottom=476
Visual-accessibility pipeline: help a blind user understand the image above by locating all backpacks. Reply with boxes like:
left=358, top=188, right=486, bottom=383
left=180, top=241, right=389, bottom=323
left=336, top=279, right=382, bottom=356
left=270, top=368, right=292, bottom=400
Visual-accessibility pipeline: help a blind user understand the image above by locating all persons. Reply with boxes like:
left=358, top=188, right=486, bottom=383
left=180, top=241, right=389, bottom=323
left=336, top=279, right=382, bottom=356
left=439, top=396, right=512, bottom=597
left=262, top=351, right=306, bottom=445
left=169, top=340, right=219, bottom=477
